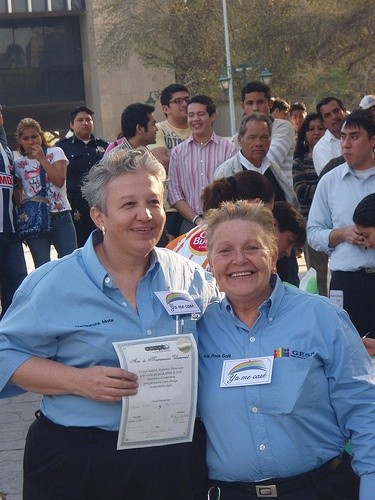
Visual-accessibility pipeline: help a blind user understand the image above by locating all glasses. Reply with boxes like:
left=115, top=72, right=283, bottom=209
left=164, top=95, right=191, bottom=106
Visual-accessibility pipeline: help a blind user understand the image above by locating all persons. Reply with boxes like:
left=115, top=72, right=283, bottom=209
left=0, top=103, right=29, bottom=323
left=292, top=113, right=328, bottom=300
left=212, top=114, right=301, bottom=290
left=103, top=102, right=170, bottom=251
left=0, top=147, right=374, bottom=499
left=318, top=156, right=347, bottom=188
left=312, top=98, right=349, bottom=179
left=192, top=202, right=375, bottom=500
left=353, top=193, right=375, bottom=362
left=164, top=202, right=305, bottom=276
left=14, top=118, right=78, bottom=271
left=173, top=171, right=276, bottom=275
left=52, top=106, right=110, bottom=254
left=144, top=84, right=193, bottom=242
left=306, top=111, right=375, bottom=340
left=358, top=95, right=375, bottom=122
left=166, top=95, right=239, bottom=237
left=230, top=79, right=294, bottom=191
left=287, top=102, right=307, bottom=259
left=268, top=99, right=290, bottom=123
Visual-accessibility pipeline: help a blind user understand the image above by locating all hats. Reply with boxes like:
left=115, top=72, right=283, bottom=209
left=359, top=94, right=375, bottom=110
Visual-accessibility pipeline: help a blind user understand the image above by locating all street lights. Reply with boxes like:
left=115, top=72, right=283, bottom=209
left=217, top=0, right=273, bottom=137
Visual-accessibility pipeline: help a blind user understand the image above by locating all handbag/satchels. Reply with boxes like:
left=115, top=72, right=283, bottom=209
left=13, top=190, right=51, bottom=241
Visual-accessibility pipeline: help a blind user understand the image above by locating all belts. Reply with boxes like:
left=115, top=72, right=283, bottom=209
left=41, top=413, right=122, bottom=444
left=209, top=456, right=342, bottom=499
left=359, top=267, right=375, bottom=276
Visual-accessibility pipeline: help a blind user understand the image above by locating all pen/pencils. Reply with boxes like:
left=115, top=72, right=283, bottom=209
left=174, top=313, right=180, bottom=336
left=181, top=317, right=185, bottom=336
